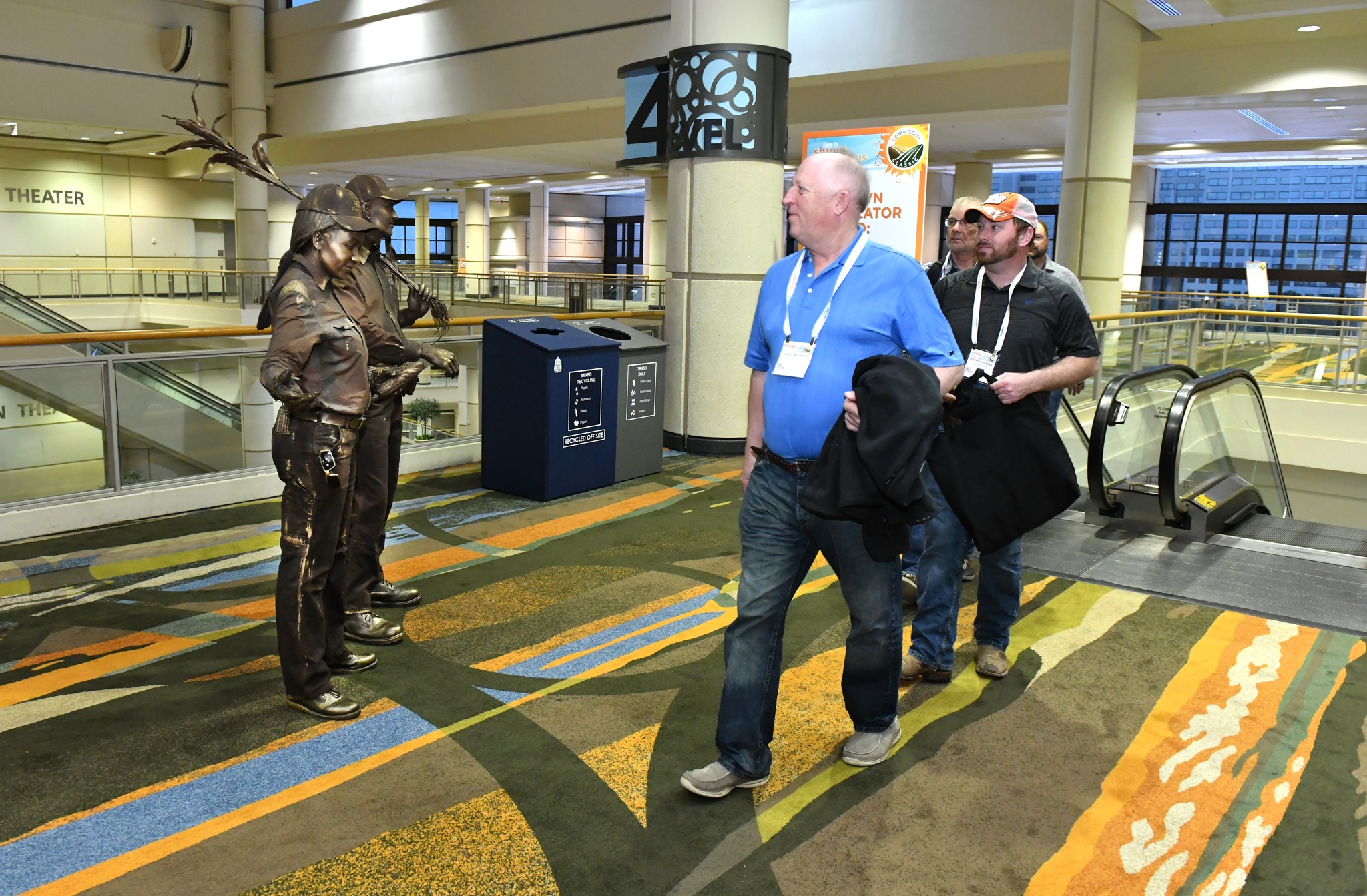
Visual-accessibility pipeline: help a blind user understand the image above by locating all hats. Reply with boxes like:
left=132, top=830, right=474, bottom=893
left=965, top=192, right=1038, bottom=225
left=346, top=174, right=410, bottom=204
left=294, top=184, right=378, bottom=233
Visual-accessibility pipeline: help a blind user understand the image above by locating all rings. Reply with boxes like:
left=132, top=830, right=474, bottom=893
left=1078, top=383, right=1082, bottom=385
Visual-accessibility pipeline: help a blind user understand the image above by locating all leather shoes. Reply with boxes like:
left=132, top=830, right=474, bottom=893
left=286, top=685, right=359, bottom=719
left=331, top=653, right=378, bottom=674
left=341, top=611, right=406, bottom=646
left=369, top=580, right=421, bottom=607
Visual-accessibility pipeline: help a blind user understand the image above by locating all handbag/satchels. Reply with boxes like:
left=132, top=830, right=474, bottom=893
left=922, top=368, right=1081, bottom=554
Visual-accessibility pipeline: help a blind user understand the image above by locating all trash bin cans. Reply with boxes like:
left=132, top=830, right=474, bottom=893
left=481, top=314, right=621, bottom=504
left=560, top=308, right=669, bottom=484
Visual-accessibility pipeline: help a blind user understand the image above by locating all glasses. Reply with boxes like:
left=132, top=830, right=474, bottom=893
left=944, top=218, right=979, bottom=229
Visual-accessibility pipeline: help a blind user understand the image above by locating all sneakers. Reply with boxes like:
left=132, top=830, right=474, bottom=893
left=962, top=558, right=975, bottom=580
left=842, top=714, right=902, bottom=767
left=900, top=572, right=918, bottom=602
left=681, top=760, right=771, bottom=798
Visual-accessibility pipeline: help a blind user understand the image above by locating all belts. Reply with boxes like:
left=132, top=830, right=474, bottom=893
left=750, top=446, right=814, bottom=473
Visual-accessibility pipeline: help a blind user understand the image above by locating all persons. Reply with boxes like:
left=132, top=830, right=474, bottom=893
left=894, top=190, right=1100, bottom=680
left=678, top=149, right=967, bottom=800
left=259, top=181, right=422, bottom=722
left=896, top=193, right=988, bottom=601
left=1021, top=219, right=1086, bottom=493
left=306, top=174, right=461, bottom=645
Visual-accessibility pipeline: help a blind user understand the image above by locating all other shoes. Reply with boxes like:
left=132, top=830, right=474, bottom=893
left=976, top=645, right=1009, bottom=677
left=899, top=654, right=953, bottom=680
